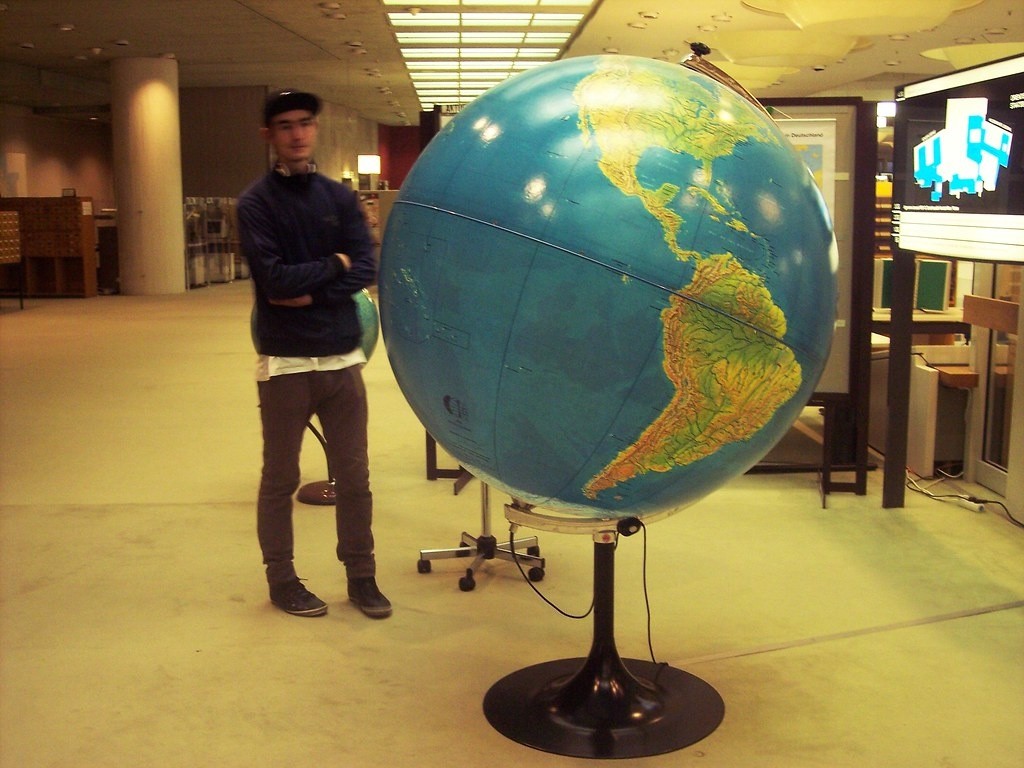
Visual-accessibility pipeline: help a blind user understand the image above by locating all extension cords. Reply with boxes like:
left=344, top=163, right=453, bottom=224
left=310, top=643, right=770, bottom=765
left=959, top=495, right=985, bottom=512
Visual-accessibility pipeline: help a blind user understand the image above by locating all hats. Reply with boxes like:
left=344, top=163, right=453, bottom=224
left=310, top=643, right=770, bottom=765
left=263, top=88, right=323, bottom=126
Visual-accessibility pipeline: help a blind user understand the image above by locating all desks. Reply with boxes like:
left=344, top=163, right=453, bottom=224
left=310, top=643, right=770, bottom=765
left=870, top=304, right=971, bottom=345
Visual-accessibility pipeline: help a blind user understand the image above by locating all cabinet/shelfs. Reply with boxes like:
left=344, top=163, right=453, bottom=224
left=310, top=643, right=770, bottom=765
left=0, top=195, right=97, bottom=310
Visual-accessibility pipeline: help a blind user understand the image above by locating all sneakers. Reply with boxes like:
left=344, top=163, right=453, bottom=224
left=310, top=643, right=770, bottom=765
left=270, top=577, right=328, bottom=616
left=347, top=576, right=392, bottom=617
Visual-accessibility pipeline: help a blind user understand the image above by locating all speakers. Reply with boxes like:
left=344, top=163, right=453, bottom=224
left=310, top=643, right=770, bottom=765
left=916, top=260, right=951, bottom=312
left=873, top=258, right=894, bottom=313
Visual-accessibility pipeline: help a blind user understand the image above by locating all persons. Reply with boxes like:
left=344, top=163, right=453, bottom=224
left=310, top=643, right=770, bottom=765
left=236, top=89, right=392, bottom=619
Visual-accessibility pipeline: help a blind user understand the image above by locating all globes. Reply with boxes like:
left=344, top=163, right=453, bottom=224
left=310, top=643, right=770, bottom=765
left=248, top=284, right=379, bottom=506
left=372, top=51, right=840, bottom=759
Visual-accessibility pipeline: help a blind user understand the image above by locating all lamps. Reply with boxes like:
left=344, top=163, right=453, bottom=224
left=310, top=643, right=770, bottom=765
left=357, top=154, right=381, bottom=190
